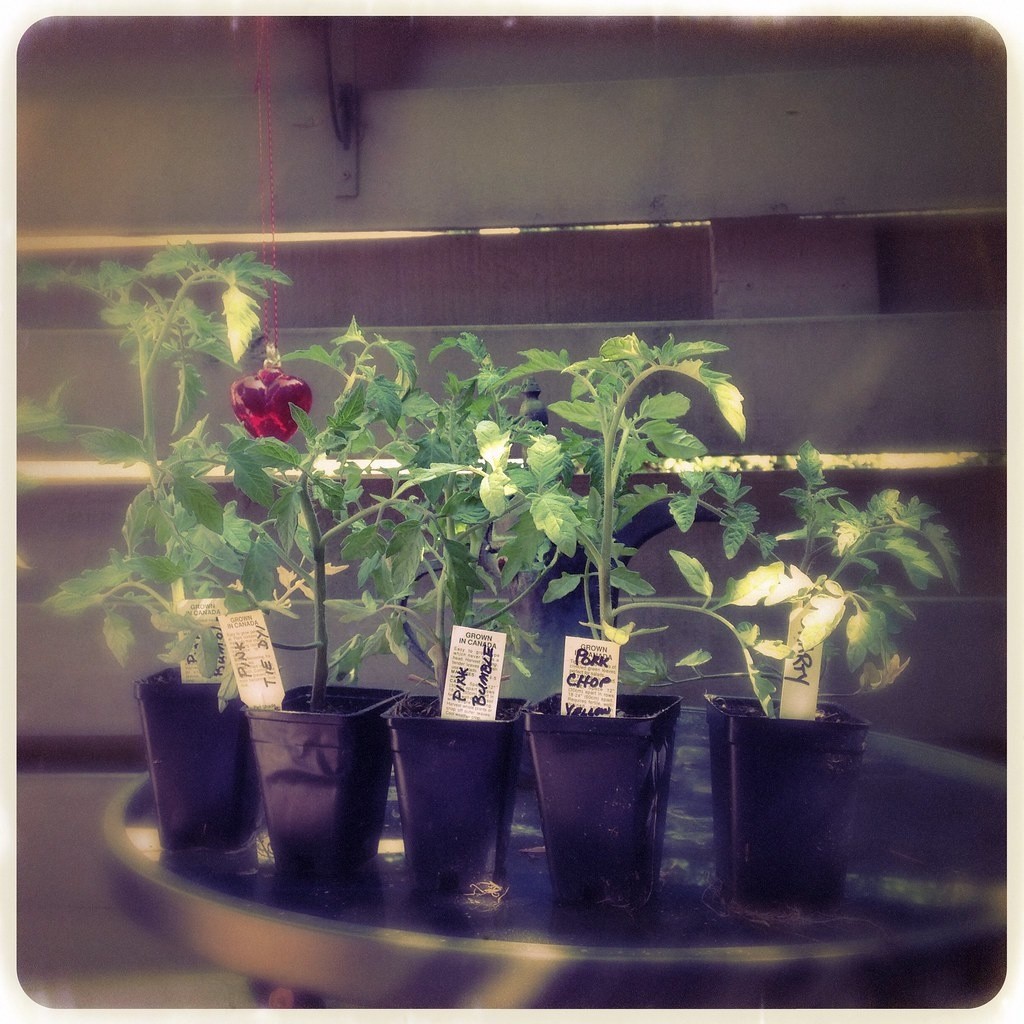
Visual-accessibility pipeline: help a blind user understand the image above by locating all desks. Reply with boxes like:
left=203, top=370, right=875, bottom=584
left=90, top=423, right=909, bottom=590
left=17, top=451, right=987, bottom=695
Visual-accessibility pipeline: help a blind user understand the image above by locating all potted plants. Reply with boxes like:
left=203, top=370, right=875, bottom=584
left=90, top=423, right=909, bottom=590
left=17, top=242, right=992, bottom=922
